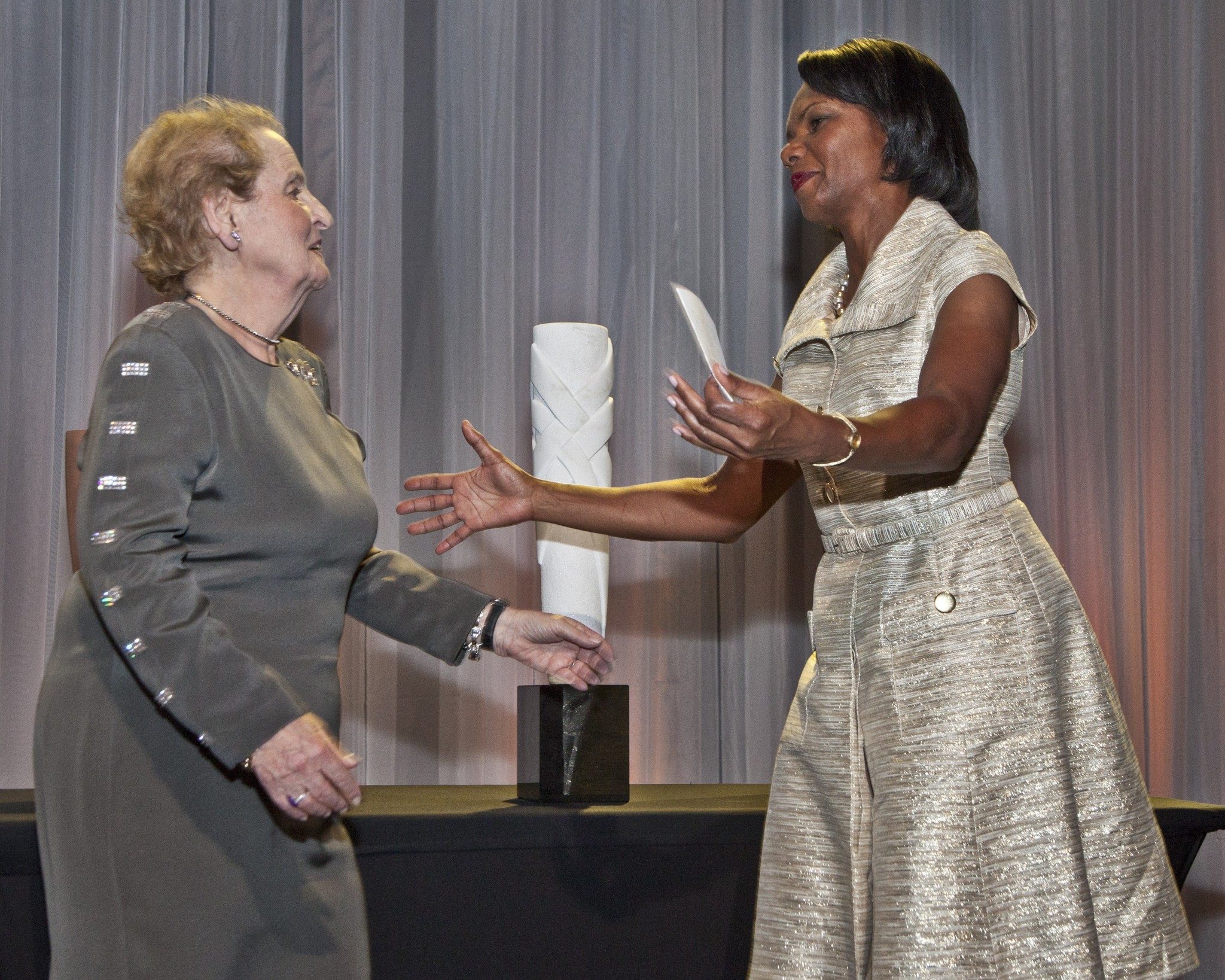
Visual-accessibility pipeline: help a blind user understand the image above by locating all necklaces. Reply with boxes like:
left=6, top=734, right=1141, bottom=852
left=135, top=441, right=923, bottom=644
left=187, top=291, right=282, bottom=347
left=833, top=269, right=848, bottom=316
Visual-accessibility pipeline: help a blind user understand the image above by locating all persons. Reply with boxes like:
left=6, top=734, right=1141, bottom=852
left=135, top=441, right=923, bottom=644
left=21, top=85, right=617, bottom=977
left=396, top=32, right=1202, bottom=979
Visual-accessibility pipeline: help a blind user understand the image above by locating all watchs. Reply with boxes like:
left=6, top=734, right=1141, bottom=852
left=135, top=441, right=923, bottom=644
left=812, top=408, right=863, bottom=468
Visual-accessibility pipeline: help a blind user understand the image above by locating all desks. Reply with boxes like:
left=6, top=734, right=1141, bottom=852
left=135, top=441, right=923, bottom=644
left=0, top=789, right=1225, bottom=980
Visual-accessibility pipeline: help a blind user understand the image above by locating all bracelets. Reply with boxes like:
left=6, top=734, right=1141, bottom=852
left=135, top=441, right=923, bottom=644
left=481, top=598, right=508, bottom=655
left=462, top=604, right=489, bottom=661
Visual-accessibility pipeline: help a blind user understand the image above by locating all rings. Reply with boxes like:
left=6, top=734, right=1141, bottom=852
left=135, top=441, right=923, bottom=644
left=291, top=790, right=309, bottom=807
